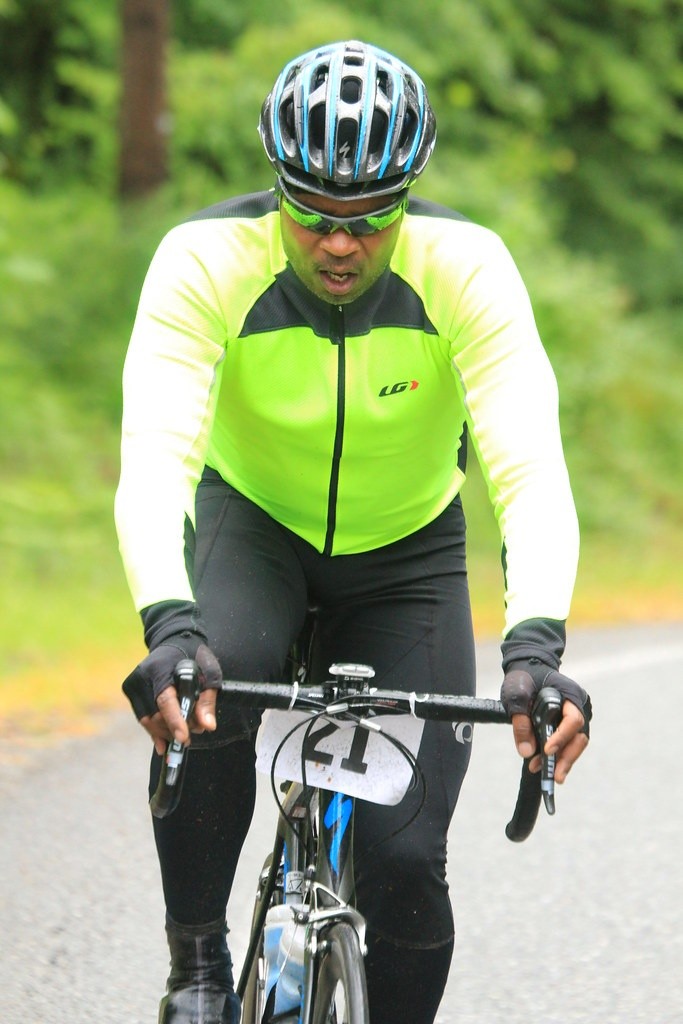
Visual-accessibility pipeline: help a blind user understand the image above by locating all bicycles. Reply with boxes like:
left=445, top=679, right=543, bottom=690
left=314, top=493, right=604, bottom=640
left=149, top=657, right=564, bottom=1023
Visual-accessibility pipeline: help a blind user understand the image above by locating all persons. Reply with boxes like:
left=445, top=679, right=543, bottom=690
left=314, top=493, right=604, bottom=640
left=112, top=40, right=593, bottom=1024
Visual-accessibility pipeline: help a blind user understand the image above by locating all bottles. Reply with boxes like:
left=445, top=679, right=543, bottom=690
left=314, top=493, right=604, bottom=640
left=264, top=870, right=309, bottom=1022
left=275, top=906, right=308, bottom=1013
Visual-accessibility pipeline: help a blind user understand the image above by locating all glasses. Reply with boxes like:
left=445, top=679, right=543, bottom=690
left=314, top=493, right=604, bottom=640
left=272, top=174, right=409, bottom=239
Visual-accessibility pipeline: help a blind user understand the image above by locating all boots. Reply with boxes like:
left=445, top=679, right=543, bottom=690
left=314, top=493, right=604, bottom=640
left=158, top=909, right=241, bottom=1024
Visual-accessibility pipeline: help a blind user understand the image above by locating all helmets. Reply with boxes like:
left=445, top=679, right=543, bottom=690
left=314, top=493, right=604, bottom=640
left=257, top=39, right=437, bottom=201
